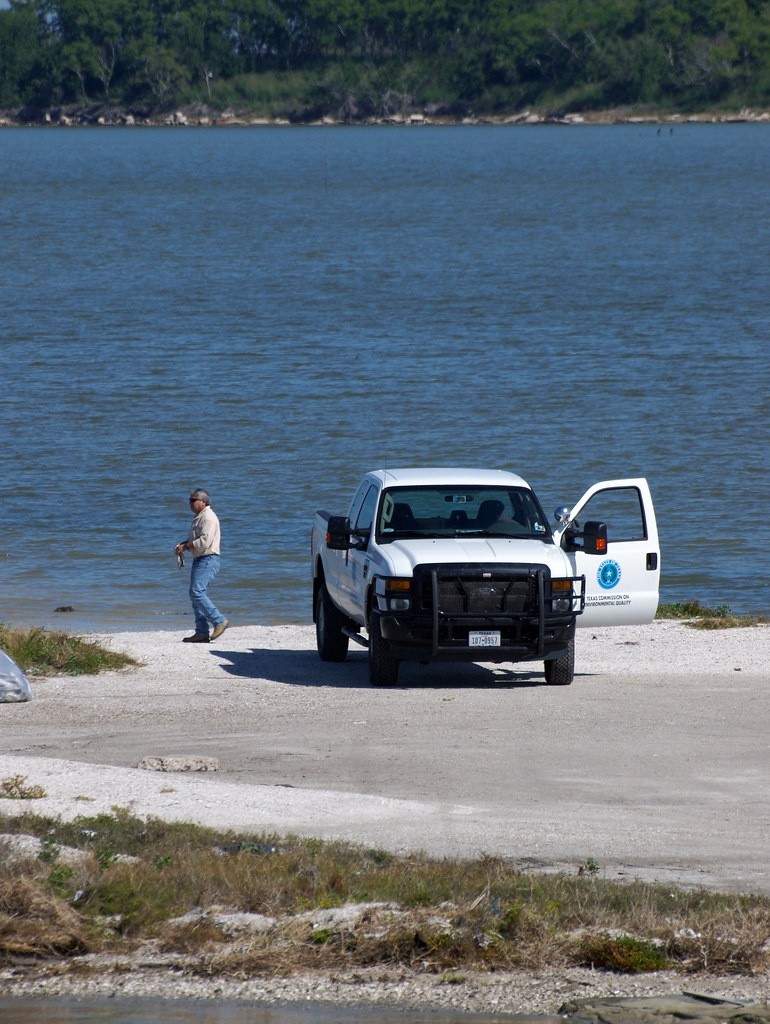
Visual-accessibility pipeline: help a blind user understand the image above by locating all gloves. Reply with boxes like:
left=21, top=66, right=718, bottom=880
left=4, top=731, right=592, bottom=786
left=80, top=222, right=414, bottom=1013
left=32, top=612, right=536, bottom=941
left=175, top=545, right=184, bottom=568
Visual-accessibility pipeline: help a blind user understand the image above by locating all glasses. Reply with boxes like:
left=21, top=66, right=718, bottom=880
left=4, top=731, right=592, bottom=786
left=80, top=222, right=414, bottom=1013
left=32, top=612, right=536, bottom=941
left=190, top=498, right=204, bottom=504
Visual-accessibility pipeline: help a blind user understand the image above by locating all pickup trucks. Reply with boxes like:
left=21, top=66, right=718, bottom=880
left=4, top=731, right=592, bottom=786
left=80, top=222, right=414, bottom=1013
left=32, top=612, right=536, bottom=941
left=309, top=468, right=661, bottom=685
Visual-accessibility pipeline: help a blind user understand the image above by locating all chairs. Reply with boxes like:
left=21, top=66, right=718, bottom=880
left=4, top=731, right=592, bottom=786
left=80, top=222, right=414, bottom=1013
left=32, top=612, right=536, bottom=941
left=369, top=503, right=417, bottom=542
left=465, top=501, right=507, bottom=532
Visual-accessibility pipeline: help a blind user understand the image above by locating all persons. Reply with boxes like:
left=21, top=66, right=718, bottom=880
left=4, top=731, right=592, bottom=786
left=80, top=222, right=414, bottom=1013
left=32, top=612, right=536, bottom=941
left=176, top=489, right=230, bottom=643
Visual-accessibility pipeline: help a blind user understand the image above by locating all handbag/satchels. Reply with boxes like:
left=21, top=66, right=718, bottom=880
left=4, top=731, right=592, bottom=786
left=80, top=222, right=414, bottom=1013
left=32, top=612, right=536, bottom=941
left=0, top=650, right=32, bottom=702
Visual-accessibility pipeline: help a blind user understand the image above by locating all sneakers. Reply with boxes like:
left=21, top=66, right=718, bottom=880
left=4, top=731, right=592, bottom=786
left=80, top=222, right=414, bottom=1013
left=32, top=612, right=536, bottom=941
left=210, top=619, right=230, bottom=640
left=183, top=633, right=210, bottom=643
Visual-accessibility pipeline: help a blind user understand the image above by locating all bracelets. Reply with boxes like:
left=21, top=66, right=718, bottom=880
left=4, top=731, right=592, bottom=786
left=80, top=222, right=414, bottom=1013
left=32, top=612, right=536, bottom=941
left=186, top=542, right=190, bottom=551
